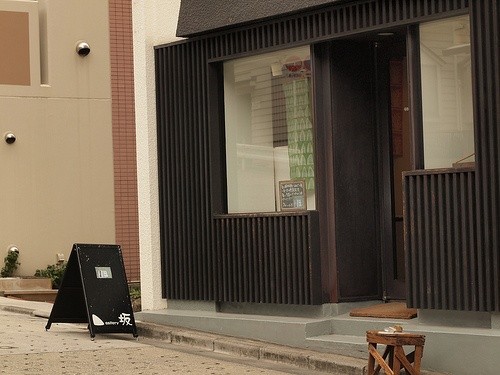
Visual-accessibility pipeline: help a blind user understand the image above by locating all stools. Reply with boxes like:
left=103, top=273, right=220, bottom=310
left=365, top=328, right=426, bottom=375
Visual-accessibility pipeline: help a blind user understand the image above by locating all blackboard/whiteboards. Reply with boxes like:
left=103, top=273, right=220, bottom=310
left=49, top=242, right=135, bottom=333
left=277, top=179, right=308, bottom=210
